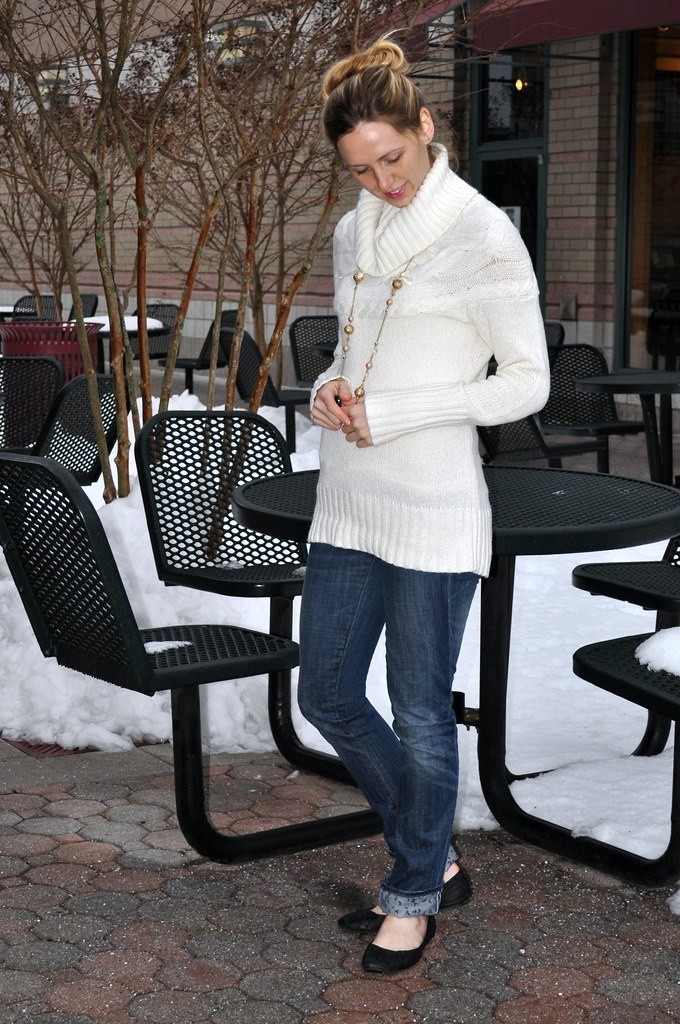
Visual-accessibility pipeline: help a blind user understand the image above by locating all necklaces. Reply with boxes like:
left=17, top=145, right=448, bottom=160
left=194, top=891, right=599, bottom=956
left=338, top=257, right=413, bottom=406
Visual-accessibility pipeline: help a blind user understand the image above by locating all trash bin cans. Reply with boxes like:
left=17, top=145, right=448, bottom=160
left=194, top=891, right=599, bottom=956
left=0, top=321, right=108, bottom=448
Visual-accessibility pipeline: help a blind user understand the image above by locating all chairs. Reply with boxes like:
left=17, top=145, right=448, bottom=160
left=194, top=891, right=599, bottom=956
left=0, top=295, right=680, bottom=886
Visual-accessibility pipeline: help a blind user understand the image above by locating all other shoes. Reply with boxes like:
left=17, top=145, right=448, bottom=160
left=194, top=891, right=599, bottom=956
left=337, top=865, right=473, bottom=933
left=362, top=914, right=436, bottom=974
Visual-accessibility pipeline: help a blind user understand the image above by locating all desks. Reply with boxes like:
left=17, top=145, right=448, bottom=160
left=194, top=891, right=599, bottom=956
left=310, top=341, right=339, bottom=358
left=97, top=320, right=174, bottom=375
left=573, top=370, right=680, bottom=484
left=167, top=467, right=680, bottom=885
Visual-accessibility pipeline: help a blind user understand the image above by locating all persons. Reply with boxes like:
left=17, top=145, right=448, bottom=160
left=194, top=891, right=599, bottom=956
left=299, top=32, right=551, bottom=972
